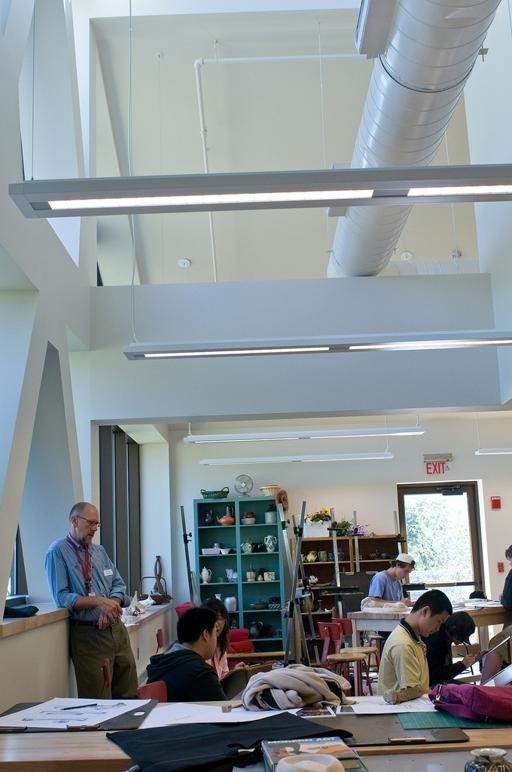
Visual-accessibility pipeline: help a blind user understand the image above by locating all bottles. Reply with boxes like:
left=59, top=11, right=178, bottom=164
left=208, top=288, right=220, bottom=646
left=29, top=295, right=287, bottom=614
left=247, top=560, right=256, bottom=582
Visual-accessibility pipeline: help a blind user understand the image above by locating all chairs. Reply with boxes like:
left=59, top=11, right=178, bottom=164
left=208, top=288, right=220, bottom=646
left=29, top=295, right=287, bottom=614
left=317, top=623, right=373, bottom=697
left=331, top=617, right=380, bottom=682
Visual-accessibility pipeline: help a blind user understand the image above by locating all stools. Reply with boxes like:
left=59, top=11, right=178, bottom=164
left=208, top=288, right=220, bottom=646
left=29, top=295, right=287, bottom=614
left=366, top=637, right=385, bottom=686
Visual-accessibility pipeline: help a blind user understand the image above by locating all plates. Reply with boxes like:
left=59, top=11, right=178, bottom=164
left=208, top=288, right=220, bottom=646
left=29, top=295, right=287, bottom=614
left=250, top=604, right=267, bottom=609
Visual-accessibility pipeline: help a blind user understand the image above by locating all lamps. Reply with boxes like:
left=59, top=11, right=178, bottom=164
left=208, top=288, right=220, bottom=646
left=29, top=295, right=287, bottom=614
left=198, top=415, right=394, bottom=467
left=8, top=0, right=510, bottom=220
left=183, top=416, right=425, bottom=446
left=116, top=0, right=512, bottom=360
left=471, top=418, right=511, bottom=455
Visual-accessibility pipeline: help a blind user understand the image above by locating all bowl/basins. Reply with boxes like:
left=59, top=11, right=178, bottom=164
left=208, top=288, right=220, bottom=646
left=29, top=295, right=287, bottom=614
left=220, top=549, right=231, bottom=554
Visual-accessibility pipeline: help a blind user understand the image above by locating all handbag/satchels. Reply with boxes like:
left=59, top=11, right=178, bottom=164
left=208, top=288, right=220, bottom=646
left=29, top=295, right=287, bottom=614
left=105, top=710, right=354, bottom=772
left=427, top=683, right=512, bottom=724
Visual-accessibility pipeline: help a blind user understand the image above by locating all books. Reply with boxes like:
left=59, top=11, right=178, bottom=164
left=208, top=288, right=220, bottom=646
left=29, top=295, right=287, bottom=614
left=262, top=735, right=368, bottom=772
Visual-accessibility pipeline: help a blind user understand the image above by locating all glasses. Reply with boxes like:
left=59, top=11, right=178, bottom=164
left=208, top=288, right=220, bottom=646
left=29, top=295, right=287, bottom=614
left=77, top=515, right=102, bottom=528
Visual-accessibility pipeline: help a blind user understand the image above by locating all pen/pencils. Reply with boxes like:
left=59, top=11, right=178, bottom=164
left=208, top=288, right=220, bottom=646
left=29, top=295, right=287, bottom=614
left=61, top=703, right=97, bottom=710
left=457, top=653, right=465, bottom=657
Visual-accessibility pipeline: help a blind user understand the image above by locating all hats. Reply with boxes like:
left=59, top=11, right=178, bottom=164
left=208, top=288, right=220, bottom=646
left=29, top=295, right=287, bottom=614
left=396, top=552, right=415, bottom=566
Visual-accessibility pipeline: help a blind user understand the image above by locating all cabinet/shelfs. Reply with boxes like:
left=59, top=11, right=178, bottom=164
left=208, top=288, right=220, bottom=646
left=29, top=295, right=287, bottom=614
left=290, top=534, right=398, bottom=656
left=193, top=496, right=297, bottom=659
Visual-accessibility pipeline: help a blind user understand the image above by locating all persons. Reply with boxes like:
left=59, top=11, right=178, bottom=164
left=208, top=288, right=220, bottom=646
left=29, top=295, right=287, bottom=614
left=44, top=501, right=139, bottom=700
left=368, top=552, right=418, bottom=607
left=480, top=625, right=512, bottom=688
left=377, top=589, right=453, bottom=696
left=146, top=606, right=252, bottom=701
left=500, top=544, right=512, bottom=630
left=198, top=597, right=229, bottom=681
left=422, top=611, right=476, bottom=691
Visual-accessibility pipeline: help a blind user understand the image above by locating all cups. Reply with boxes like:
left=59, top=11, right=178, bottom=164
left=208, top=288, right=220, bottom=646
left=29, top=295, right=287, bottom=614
left=318, top=551, right=327, bottom=562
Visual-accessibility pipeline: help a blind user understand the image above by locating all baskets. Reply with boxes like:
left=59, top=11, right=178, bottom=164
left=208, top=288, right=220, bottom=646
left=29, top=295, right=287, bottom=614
left=200, top=486, right=230, bottom=498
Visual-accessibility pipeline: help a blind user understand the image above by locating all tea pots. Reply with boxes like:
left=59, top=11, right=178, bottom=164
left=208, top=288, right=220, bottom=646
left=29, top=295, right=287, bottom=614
left=304, top=552, right=317, bottom=562
left=216, top=515, right=235, bottom=526
left=200, top=566, right=212, bottom=583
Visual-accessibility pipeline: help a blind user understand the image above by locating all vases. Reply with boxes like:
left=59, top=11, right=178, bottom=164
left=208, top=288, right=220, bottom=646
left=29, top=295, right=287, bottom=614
left=303, top=523, right=330, bottom=537
left=264, top=535, right=277, bottom=553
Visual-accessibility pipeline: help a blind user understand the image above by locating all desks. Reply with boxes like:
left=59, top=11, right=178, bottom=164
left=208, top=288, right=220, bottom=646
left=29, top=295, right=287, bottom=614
left=347, top=599, right=505, bottom=696
left=1, top=702, right=512, bottom=771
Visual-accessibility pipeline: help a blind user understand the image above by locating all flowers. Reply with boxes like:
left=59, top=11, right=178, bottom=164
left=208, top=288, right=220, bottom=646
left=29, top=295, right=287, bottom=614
left=305, top=506, right=331, bottom=522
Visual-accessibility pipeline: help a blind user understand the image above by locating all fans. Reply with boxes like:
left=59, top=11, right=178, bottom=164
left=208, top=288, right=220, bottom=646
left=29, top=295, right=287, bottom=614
left=235, top=474, right=254, bottom=497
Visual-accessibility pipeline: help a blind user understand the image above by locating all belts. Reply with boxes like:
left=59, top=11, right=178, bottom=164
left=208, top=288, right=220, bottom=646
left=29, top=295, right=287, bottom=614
left=69, top=617, right=120, bottom=626
